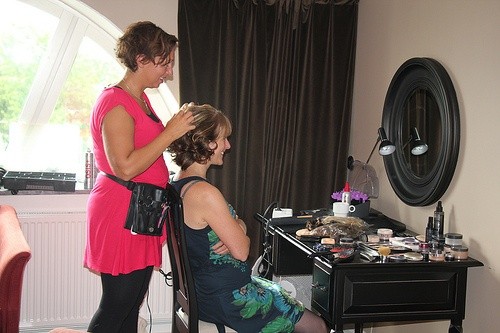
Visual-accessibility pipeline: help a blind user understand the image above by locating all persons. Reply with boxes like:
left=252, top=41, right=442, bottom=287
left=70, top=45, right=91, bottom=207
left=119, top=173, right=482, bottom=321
left=166, top=104, right=327, bottom=333
left=83, top=22, right=196, bottom=333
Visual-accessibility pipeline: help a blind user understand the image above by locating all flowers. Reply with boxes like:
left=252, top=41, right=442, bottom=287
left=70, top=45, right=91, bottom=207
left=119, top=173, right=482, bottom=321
left=330, top=187, right=369, bottom=204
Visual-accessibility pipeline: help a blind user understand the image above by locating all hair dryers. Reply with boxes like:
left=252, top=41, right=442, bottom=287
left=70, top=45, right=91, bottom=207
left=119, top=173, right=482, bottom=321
left=269, top=208, right=334, bottom=227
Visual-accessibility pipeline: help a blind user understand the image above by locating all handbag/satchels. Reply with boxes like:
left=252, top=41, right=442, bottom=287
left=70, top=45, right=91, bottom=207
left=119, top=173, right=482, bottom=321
left=123, top=182, right=168, bottom=236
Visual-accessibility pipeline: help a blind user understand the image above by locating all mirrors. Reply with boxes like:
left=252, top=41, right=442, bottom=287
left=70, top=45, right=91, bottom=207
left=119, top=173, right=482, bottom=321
left=380, top=57, right=462, bottom=208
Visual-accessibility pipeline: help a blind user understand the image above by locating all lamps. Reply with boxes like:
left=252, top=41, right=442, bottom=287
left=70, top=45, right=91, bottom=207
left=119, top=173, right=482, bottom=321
left=365, top=127, right=395, bottom=165
left=400, top=127, right=428, bottom=157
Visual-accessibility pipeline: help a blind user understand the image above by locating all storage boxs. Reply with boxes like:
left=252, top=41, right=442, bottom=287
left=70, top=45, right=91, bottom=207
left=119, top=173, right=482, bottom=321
left=332, top=200, right=370, bottom=219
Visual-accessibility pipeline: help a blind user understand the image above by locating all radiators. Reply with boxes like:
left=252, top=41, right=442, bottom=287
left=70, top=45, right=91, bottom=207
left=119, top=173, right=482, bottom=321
left=18, top=210, right=174, bottom=333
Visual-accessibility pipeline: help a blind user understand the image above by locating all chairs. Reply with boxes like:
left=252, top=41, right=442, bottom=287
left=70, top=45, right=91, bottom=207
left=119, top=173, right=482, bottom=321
left=164, top=182, right=241, bottom=333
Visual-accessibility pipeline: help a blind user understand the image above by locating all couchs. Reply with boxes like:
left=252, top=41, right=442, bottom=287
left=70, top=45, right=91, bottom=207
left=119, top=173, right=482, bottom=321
left=0, top=205, right=34, bottom=333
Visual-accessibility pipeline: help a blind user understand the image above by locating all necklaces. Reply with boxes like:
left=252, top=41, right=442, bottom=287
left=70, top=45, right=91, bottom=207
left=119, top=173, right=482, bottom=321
left=123, top=79, right=149, bottom=111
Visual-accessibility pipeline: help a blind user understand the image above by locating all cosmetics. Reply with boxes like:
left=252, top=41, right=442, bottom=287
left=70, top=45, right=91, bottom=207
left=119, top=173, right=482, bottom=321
left=296, top=182, right=468, bottom=263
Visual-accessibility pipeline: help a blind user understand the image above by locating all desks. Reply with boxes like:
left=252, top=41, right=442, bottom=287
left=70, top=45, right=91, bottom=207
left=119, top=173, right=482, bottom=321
left=253, top=209, right=485, bottom=333
